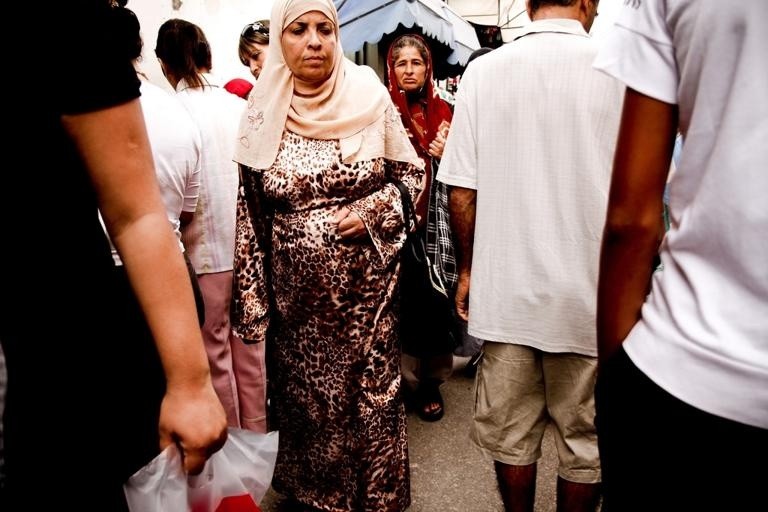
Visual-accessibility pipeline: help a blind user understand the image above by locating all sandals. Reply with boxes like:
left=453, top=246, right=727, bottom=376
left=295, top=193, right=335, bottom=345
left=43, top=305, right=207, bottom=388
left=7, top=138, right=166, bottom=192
left=409, top=376, right=444, bottom=422
left=463, top=352, right=483, bottom=378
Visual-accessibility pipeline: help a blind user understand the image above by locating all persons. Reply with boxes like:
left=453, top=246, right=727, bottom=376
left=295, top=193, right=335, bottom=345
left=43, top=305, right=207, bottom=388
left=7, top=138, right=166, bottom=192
left=238, top=19, right=271, bottom=80
left=1, top=1, right=230, bottom=511
left=436, top=1, right=626, bottom=512
left=386, top=30, right=466, bottom=423
left=455, top=46, right=496, bottom=378
left=230, top=1, right=426, bottom=512
left=155, top=18, right=270, bottom=435
left=593, top=1, right=768, bottom=512
left=98, top=6, right=206, bottom=472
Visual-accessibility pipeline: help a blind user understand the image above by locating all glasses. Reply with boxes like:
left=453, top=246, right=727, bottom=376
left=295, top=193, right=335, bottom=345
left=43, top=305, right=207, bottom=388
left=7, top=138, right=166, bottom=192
left=241, top=23, right=269, bottom=35
